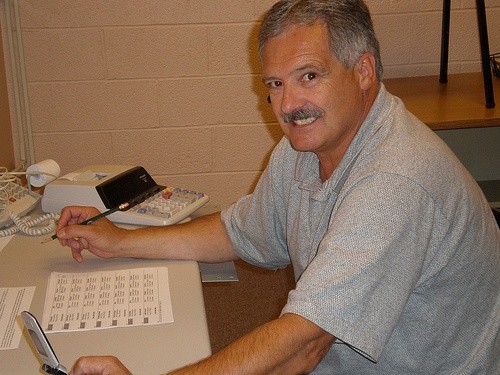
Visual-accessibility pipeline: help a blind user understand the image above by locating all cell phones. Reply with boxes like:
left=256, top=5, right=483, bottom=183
left=20, top=311, right=68, bottom=375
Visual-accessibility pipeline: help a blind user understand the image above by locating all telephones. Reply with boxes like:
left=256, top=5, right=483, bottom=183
left=0, top=176, right=60, bottom=238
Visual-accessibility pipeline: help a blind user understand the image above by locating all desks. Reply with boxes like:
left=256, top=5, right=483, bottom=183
left=379, top=71, right=499, bottom=212
left=0, top=202, right=213, bottom=375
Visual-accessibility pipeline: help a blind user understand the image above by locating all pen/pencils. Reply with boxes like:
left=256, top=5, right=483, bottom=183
left=40, top=201, right=129, bottom=244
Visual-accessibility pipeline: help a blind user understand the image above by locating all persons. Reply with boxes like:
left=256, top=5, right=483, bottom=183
left=53, top=0, right=500, bottom=375
